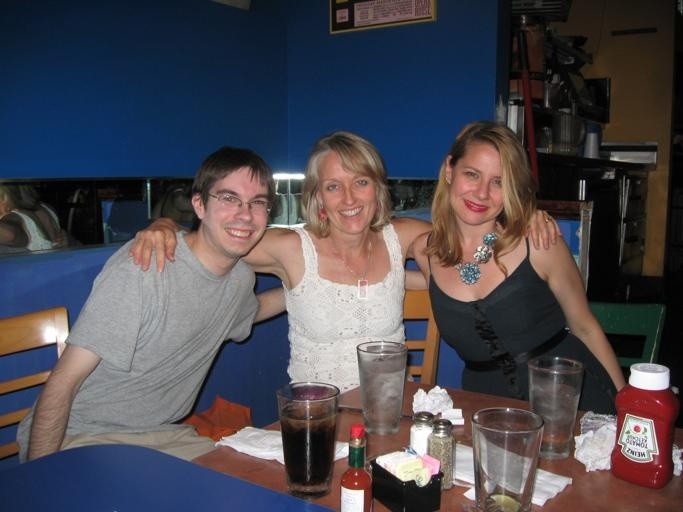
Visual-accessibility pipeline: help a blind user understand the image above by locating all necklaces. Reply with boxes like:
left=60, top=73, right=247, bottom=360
left=437, top=221, right=500, bottom=285
left=325, top=233, right=372, bottom=302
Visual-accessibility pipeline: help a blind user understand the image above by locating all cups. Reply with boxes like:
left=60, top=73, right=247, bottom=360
left=276, top=380, right=340, bottom=499
left=582, top=134, right=601, bottom=159
left=357, top=340, right=409, bottom=435
left=527, top=355, right=585, bottom=460
left=470, top=407, right=544, bottom=512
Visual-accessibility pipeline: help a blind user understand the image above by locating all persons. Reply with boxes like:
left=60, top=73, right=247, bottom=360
left=401, top=120, right=626, bottom=419
left=131, top=130, right=562, bottom=405
left=18, top=147, right=287, bottom=465
left=0, top=184, right=67, bottom=248
left=153, top=177, right=193, bottom=225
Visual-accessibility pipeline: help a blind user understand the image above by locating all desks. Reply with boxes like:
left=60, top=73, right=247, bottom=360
left=187, top=377, right=683, bottom=512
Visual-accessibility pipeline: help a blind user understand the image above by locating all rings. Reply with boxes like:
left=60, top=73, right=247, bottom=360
left=542, top=216, right=552, bottom=223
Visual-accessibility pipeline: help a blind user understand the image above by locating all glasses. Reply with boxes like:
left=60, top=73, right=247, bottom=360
left=206, top=191, right=273, bottom=213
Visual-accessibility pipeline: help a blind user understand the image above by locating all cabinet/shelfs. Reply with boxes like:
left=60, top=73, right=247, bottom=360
left=539, top=146, right=655, bottom=282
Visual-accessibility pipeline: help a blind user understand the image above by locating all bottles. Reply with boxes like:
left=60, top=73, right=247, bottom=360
left=410, top=413, right=434, bottom=458
left=340, top=425, right=372, bottom=512
left=427, top=419, right=456, bottom=491
left=611, top=363, right=679, bottom=490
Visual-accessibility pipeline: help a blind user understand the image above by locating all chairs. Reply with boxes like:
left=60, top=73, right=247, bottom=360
left=585, top=300, right=666, bottom=377
left=401, top=288, right=441, bottom=386
left=0, top=307, right=72, bottom=464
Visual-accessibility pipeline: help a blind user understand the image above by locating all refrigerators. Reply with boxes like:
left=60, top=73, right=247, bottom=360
left=538, top=153, right=648, bottom=307
